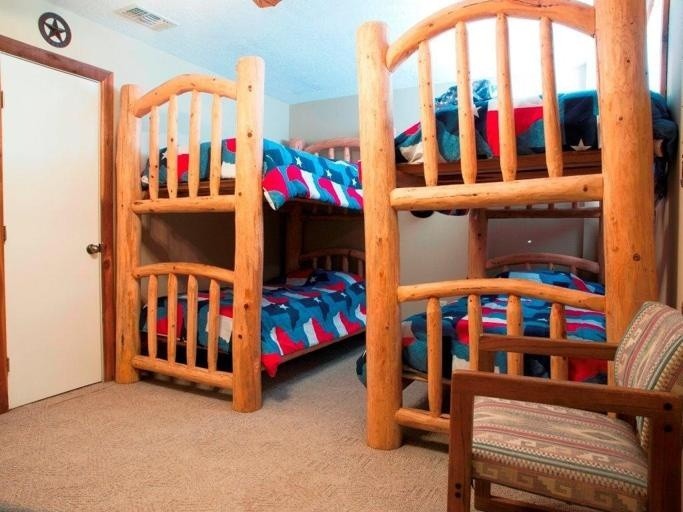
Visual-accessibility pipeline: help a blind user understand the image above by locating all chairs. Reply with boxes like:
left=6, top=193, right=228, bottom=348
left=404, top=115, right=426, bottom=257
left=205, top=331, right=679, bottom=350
left=447, top=302, right=683, bottom=510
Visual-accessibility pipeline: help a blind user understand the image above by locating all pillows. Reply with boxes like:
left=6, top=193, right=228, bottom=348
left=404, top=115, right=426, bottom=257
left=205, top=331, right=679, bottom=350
left=283, top=265, right=365, bottom=293
left=489, top=268, right=591, bottom=303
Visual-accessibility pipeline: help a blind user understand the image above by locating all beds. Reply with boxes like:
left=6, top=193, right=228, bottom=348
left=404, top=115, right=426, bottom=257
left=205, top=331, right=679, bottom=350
left=356, top=0, right=677, bottom=450
left=112, top=54, right=367, bottom=413
